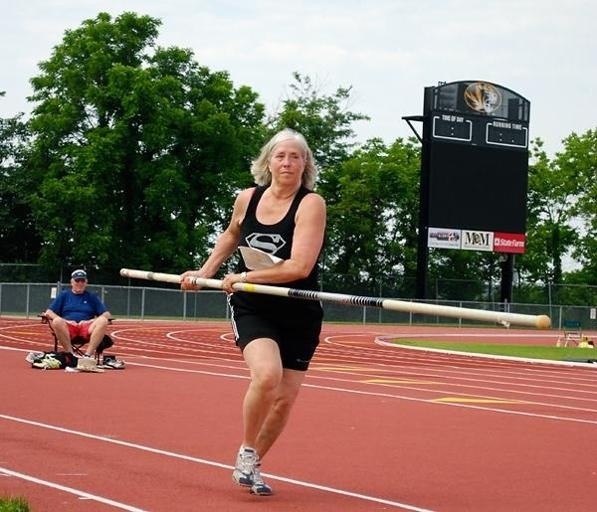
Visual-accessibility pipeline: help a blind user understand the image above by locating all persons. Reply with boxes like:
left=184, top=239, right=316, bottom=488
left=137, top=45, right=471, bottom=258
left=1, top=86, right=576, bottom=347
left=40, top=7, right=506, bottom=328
left=179, top=126, right=328, bottom=496
left=45, top=270, right=111, bottom=359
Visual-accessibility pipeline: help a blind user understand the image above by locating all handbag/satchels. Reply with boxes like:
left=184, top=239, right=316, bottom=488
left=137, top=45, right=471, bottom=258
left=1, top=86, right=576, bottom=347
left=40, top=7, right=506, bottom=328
left=32, top=352, right=77, bottom=369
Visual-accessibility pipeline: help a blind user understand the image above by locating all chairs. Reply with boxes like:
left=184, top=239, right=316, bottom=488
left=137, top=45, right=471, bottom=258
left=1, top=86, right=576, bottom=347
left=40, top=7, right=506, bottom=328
left=37, top=311, right=115, bottom=365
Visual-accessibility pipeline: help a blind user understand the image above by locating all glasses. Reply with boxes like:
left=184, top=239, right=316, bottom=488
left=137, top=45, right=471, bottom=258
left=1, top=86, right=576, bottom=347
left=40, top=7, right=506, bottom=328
left=75, top=279, right=85, bottom=283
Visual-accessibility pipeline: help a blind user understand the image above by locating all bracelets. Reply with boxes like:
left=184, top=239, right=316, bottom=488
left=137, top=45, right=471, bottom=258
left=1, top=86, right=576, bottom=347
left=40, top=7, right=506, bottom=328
left=241, top=272, right=247, bottom=283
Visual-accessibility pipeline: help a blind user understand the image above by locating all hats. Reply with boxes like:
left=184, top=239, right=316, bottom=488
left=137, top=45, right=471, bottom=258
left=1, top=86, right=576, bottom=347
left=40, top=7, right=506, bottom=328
left=72, top=270, right=86, bottom=279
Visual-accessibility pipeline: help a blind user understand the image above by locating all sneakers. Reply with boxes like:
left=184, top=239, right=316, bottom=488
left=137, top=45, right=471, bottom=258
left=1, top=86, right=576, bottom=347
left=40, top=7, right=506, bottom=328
left=232, top=445, right=272, bottom=495
